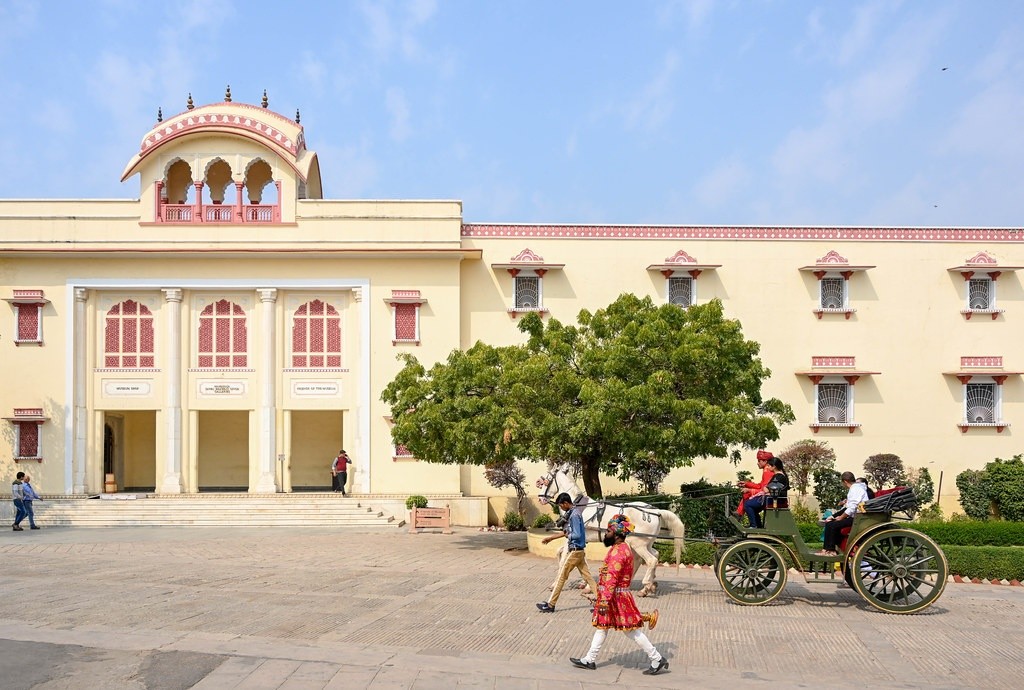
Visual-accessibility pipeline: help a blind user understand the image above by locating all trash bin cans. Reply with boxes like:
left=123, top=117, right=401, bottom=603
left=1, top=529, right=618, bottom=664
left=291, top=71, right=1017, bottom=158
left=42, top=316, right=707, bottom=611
left=331, top=472, right=338, bottom=491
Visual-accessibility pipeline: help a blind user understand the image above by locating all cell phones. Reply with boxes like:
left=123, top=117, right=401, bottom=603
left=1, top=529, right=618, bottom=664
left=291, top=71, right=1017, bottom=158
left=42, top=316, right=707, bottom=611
left=345, top=454, right=347, bottom=456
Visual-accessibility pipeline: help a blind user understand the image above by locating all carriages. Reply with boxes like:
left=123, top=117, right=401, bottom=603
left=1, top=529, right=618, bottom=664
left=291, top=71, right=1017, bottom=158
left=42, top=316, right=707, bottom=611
left=537, top=462, right=949, bottom=614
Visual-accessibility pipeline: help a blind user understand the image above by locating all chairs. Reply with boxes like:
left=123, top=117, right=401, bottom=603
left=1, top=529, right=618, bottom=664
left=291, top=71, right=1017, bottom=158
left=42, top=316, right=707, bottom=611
left=760, top=489, right=911, bottom=554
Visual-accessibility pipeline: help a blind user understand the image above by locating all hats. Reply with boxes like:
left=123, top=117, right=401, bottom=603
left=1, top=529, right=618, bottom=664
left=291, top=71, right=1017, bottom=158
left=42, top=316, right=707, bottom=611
left=609, top=514, right=635, bottom=537
left=757, top=451, right=774, bottom=460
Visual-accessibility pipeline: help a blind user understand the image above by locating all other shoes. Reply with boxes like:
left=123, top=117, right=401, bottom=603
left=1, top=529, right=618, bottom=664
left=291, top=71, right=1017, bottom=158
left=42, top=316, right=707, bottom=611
left=814, top=547, right=837, bottom=556
left=341, top=492, right=346, bottom=496
left=570, top=657, right=596, bottom=670
left=837, top=581, right=850, bottom=588
left=743, top=524, right=764, bottom=530
left=31, top=526, right=40, bottom=529
left=817, top=520, right=827, bottom=527
left=12, top=523, right=20, bottom=530
left=13, top=527, right=23, bottom=531
left=728, top=509, right=742, bottom=522
left=642, top=657, right=667, bottom=675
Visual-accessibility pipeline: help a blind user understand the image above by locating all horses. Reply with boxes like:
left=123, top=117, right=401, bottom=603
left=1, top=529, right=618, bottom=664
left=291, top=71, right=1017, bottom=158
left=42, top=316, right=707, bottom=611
left=537, top=461, right=687, bottom=598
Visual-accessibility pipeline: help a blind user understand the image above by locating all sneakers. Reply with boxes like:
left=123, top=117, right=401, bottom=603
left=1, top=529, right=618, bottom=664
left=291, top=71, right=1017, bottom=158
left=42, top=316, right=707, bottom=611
left=536, top=600, right=555, bottom=613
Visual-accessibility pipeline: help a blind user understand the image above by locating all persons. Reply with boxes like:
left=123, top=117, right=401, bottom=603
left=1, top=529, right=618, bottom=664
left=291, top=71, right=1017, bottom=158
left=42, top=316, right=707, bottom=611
left=11, top=472, right=42, bottom=531
left=570, top=514, right=669, bottom=675
left=331, top=450, right=352, bottom=496
left=729, top=450, right=789, bottom=529
left=815, top=471, right=873, bottom=556
left=535, top=493, right=597, bottom=613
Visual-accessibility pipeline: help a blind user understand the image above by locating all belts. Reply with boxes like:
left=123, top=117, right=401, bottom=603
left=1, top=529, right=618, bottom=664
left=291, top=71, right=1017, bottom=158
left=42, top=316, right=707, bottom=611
left=570, top=547, right=584, bottom=551
